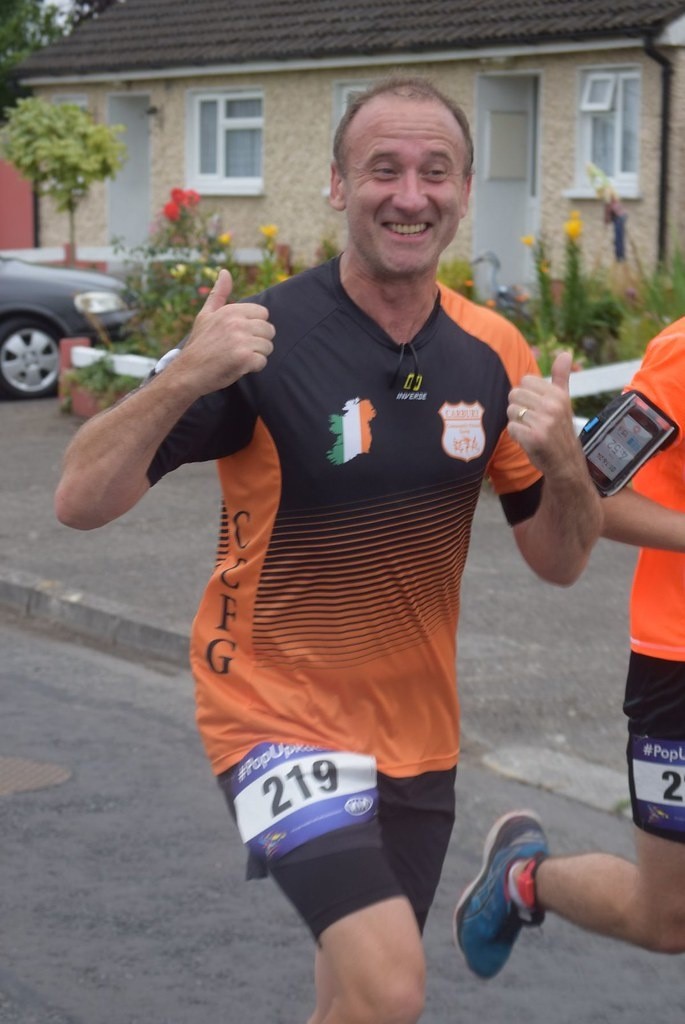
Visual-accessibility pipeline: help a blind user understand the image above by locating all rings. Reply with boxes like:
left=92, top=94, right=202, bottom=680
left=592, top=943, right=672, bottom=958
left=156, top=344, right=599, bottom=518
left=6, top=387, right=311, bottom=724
left=515, top=406, right=528, bottom=419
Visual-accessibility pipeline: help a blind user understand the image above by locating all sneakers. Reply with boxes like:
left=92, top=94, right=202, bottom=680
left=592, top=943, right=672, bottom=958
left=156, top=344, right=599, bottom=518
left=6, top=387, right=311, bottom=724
left=453, top=810, right=550, bottom=980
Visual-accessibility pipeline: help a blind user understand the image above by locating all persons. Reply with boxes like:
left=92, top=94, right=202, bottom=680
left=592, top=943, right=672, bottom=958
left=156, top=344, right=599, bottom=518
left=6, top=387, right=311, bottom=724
left=53, top=81, right=685, bottom=1023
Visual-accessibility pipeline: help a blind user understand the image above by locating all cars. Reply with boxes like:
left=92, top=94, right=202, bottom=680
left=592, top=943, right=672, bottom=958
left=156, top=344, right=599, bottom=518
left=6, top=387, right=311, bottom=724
left=1, top=257, right=140, bottom=401
